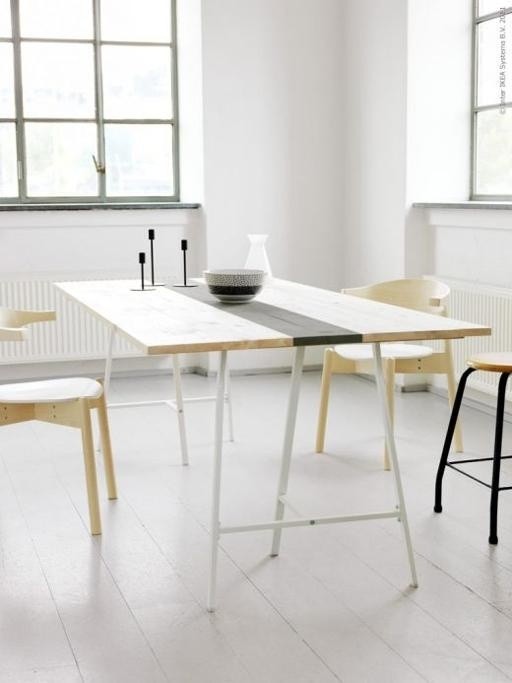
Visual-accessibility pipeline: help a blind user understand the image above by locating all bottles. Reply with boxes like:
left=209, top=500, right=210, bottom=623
left=245, top=232, right=273, bottom=285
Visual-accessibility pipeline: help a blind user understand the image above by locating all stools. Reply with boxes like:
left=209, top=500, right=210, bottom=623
left=430, top=351, right=512, bottom=545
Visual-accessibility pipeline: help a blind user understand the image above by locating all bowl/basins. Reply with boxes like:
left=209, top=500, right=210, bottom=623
left=203, top=266, right=269, bottom=304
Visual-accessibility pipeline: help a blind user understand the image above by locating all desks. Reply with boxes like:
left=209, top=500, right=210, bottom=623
left=53, top=274, right=494, bottom=612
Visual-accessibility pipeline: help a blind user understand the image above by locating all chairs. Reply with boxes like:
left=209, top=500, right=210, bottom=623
left=2, top=304, right=117, bottom=534
left=312, top=276, right=463, bottom=457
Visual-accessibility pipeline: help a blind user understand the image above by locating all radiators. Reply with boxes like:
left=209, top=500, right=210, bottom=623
left=437, top=278, right=512, bottom=400
left=2, top=272, right=167, bottom=361
